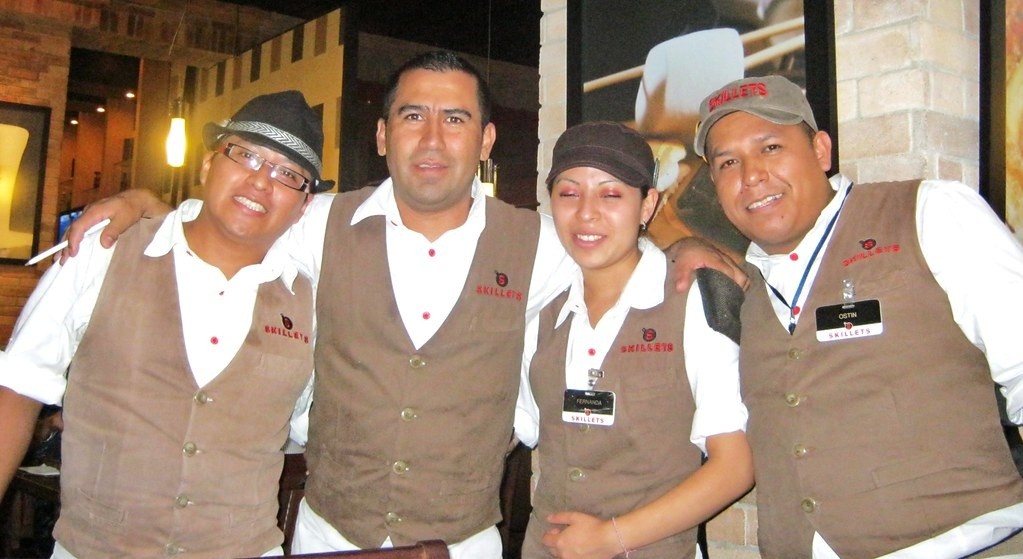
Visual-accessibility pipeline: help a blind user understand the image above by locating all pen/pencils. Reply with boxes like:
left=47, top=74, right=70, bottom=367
left=24, top=218, right=112, bottom=266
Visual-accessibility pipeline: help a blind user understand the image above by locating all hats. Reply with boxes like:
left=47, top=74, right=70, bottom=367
left=693, top=74, right=819, bottom=157
left=545, top=121, right=655, bottom=188
left=202, top=90, right=336, bottom=194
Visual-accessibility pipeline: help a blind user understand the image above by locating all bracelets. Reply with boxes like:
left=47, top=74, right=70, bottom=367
left=612, top=518, right=636, bottom=558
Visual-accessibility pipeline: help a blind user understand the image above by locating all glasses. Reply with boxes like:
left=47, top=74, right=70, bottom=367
left=214, top=142, right=310, bottom=192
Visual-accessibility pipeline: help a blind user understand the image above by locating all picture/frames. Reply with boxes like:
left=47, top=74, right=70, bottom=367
left=978, top=0, right=1022, bottom=450
left=0, top=101, right=52, bottom=265
left=566, top=0, right=839, bottom=265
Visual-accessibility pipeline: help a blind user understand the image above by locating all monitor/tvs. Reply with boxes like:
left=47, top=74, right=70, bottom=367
left=59, top=205, right=87, bottom=244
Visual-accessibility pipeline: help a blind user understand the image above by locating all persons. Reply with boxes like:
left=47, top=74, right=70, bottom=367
left=0, top=91, right=334, bottom=559
left=501, top=119, right=756, bottom=559
left=694, top=74, right=1023, bottom=559
left=52, top=49, right=751, bottom=559
